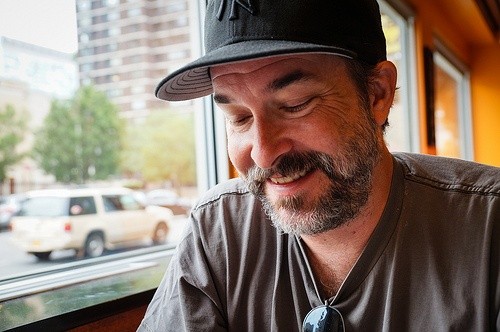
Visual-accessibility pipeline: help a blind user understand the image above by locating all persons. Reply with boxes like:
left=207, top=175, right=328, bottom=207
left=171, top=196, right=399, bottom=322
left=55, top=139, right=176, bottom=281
left=134, top=0, right=500, bottom=332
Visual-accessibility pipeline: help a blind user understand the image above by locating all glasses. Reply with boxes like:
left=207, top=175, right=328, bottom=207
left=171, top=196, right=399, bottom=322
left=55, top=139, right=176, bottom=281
left=303, top=300, right=345, bottom=332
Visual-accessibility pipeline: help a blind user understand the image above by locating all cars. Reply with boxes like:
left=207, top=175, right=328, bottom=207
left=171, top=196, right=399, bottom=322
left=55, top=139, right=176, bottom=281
left=10, top=186, right=175, bottom=259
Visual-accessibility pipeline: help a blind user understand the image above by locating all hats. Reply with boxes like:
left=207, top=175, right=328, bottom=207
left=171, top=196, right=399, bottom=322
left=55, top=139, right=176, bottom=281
left=155, top=0, right=386, bottom=101
